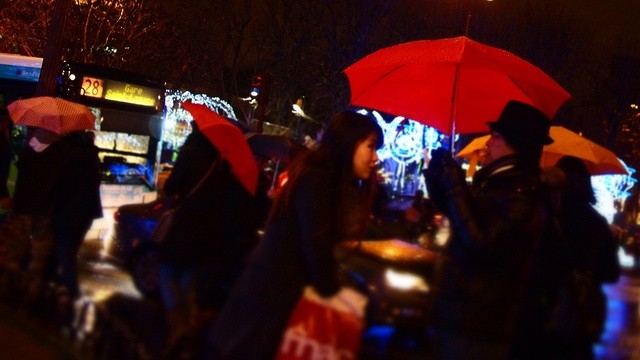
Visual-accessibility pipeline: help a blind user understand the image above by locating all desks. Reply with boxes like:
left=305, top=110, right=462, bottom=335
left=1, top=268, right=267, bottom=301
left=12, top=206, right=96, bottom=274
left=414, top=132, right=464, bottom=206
left=341, top=236, right=439, bottom=289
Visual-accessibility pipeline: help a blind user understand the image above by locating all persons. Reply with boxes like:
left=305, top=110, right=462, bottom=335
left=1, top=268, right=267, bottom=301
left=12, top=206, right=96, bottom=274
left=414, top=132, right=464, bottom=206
left=553, top=149, right=620, bottom=359
left=1, top=107, right=15, bottom=209
left=38, top=131, right=102, bottom=315
left=160, top=123, right=234, bottom=282
left=220, top=109, right=385, bottom=360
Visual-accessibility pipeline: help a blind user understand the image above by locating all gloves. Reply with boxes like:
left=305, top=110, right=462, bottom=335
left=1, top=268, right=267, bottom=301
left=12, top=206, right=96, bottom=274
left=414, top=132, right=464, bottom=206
left=422, top=147, right=466, bottom=202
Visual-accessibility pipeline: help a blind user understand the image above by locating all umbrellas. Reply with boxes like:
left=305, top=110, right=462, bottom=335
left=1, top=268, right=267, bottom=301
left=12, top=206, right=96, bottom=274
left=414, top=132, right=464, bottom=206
left=456, top=121, right=632, bottom=186
left=418, top=97, right=583, bottom=360
left=340, top=30, right=574, bottom=184
left=177, top=99, right=262, bottom=198
left=7, top=92, right=99, bottom=139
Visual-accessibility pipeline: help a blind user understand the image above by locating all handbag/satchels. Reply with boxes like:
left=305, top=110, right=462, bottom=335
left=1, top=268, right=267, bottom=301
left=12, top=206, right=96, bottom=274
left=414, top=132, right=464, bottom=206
left=149, top=208, right=175, bottom=243
left=573, top=269, right=605, bottom=344
left=275, top=285, right=368, bottom=360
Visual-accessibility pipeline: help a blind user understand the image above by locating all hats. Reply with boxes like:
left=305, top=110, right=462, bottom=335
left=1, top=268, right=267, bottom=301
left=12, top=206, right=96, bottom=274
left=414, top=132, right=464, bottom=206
left=484, top=98, right=555, bottom=145
